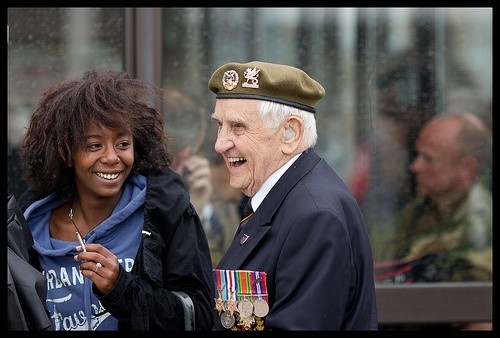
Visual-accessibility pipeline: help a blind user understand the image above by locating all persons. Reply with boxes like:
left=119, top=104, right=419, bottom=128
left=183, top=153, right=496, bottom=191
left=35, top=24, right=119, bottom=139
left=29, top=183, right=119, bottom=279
left=401, top=110, right=492, bottom=283
left=7, top=68, right=216, bottom=334
left=203, top=61, right=378, bottom=333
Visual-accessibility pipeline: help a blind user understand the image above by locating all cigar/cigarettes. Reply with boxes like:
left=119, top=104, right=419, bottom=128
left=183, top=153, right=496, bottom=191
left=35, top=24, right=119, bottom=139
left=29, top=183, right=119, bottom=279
left=75, top=231, right=87, bottom=253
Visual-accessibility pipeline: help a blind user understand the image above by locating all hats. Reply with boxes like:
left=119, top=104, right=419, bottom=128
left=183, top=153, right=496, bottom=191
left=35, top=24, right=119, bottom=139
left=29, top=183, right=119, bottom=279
left=209, top=60, right=325, bottom=113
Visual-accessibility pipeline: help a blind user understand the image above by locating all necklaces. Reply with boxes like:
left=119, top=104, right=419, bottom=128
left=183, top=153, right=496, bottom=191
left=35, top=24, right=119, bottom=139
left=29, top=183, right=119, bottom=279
left=67, top=197, right=94, bottom=243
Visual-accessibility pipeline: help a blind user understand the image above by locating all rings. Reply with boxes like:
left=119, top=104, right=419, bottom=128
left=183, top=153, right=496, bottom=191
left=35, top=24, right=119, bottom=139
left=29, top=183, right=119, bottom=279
left=93, top=263, right=102, bottom=273
